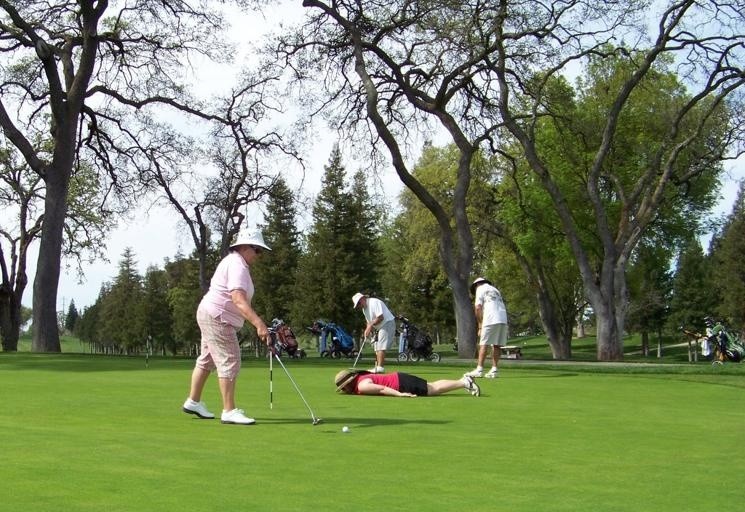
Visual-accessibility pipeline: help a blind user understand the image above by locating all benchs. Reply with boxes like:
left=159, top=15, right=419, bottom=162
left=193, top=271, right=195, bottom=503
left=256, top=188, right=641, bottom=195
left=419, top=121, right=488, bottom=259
left=499, top=346, right=523, bottom=360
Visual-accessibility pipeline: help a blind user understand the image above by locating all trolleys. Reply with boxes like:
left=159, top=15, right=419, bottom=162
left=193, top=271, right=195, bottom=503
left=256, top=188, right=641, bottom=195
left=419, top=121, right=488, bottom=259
left=266, top=317, right=306, bottom=360
left=316, top=321, right=361, bottom=360
left=679, top=315, right=745, bottom=366
left=394, top=315, right=441, bottom=362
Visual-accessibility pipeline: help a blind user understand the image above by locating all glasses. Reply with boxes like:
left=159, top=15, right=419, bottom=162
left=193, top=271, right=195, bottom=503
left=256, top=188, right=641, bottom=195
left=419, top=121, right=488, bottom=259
left=248, top=244, right=261, bottom=254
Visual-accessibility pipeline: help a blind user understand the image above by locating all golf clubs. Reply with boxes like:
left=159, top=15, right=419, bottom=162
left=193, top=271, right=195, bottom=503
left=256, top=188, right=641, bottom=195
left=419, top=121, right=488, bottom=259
left=349, top=338, right=366, bottom=370
left=265, top=339, right=323, bottom=424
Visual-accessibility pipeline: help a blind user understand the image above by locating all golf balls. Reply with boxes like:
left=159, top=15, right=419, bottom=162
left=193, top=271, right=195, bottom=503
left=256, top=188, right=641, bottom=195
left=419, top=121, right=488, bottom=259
left=342, top=426, right=349, bottom=432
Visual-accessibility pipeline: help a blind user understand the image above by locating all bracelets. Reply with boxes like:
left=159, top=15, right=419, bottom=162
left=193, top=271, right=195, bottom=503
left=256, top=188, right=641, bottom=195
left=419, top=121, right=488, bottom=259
left=478, top=327, right=481, bottom=330
left=370, top=321, right=375, bottom=327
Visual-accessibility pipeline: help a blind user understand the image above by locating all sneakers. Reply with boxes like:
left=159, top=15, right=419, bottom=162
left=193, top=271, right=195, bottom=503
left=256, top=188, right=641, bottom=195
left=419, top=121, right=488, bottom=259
left=466, top=370, right=482, bottom=377
left=183, top=400, right=215, bottom=419
left=485, top=369, right=498, bottom=378
left=221, top=407, right=256, bottom=424
left=462, top=375, right=480, bottom=397
left=367, top=365, right=385, bottom=373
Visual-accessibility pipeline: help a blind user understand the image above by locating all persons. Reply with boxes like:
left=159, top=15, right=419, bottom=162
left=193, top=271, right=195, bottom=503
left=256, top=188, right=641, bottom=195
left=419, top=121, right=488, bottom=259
left=463, top=276, right=509, bottom=379
left=181, top=227, right=274, bottom=423
left=334, top=369, right=482, bottom=398
left=352, top=292, right=398, bottom=374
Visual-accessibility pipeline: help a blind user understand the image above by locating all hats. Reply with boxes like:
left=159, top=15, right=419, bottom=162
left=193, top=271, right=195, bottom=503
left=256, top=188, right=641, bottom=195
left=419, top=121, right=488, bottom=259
left=229, top=229, right=273, bottom=251
left=470, top=277, right=491, bottom=295
left=335, top=371, right=359, bottom=392
left=352, top=292, right=369, bottom=308
